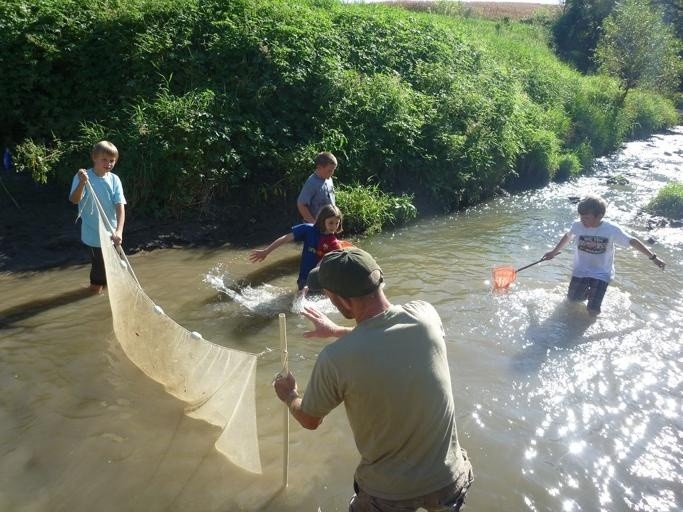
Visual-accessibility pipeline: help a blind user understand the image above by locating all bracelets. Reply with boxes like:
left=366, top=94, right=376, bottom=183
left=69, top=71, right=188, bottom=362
left=649, top=253, right=657, bottom=260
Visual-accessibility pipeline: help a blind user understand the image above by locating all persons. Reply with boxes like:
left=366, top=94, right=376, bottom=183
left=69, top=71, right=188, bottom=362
left=274, top=248, right=475, bottom=512
left=248, top=151, right=344, bottom=296
left=542, top=196, right=667, bottom=313
left=68, top=141, right=128, bottom=296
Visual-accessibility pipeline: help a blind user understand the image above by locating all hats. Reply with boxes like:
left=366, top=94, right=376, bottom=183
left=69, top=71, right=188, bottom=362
left=307, top=248, right=385, bottom=298
